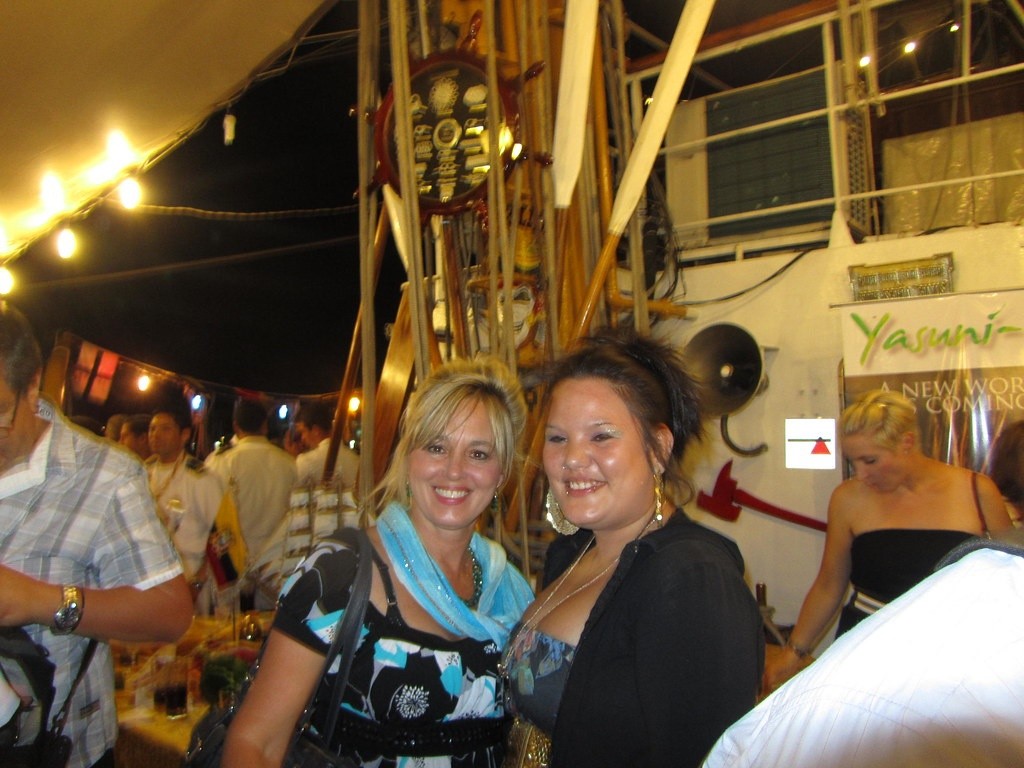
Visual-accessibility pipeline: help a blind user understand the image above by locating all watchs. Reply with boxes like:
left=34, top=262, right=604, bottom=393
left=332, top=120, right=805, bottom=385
left=51, top=585, right=80, bottom=634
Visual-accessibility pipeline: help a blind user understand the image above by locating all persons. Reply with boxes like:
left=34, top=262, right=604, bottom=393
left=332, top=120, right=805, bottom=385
left=703, top=387, right=1024, bottom=768
left=218, top=373, right=535, bottom=768
left=499, top=323, right=763, bottom=768
left=0, top=299, right=194, bottom=767
left=71, top=394, right=360, bottom=618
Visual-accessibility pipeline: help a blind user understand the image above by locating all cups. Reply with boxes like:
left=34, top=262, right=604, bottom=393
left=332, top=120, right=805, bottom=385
left=150, top=656, right=188, bottom=720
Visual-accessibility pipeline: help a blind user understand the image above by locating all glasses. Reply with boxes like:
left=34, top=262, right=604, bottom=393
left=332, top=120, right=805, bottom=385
left=0, top=389, right=22, bottom=441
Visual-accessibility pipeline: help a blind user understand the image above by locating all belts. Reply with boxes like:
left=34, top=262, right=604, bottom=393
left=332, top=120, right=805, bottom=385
left=346, top=720, right=503, bottom=759
left=506, top=716, right=551, bottom=767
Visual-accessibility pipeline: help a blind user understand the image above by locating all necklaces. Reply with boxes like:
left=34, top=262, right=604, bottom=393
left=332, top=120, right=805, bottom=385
left=409, top=544, right=483, bottom=609
left=500, top=495, right=667, bottom=676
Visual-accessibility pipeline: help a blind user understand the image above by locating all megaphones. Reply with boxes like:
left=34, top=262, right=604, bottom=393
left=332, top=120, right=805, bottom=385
left=682, top=322, right=769, bottom=419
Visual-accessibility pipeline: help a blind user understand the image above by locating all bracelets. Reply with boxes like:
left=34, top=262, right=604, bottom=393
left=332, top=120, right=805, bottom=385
left=70, top=588, right=85, bottom=632
left=787, top=641, right=804, bottom=657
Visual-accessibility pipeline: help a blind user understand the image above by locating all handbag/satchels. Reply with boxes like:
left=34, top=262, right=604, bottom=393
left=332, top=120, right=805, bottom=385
left=185, top=525, right=373, bottom=768
left=1, top=623, right=100, bottom=767
